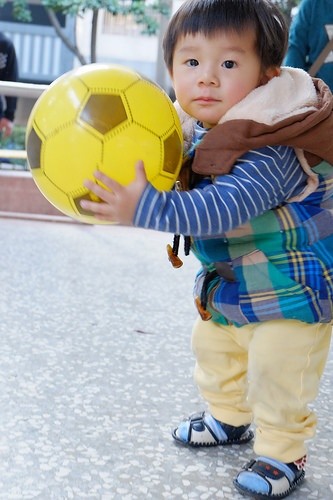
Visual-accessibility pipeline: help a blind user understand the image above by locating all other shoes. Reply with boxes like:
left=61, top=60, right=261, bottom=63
left=171, top=410, right=254, bottom=448
left=232, top=454, right=308, bottom=500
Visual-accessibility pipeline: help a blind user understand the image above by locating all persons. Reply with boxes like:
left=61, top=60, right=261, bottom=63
left=283, top=0, right=333, bottom=95
left=0, top=32, right=19, bottom=136
left=79, top=0, right=333, bottom=500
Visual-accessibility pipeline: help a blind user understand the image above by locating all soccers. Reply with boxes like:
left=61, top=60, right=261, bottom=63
left=25, top=63, right=185, bottom=224
